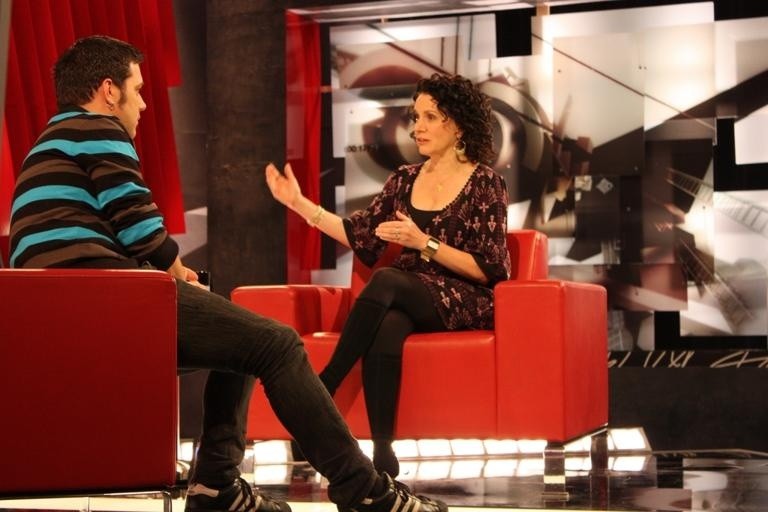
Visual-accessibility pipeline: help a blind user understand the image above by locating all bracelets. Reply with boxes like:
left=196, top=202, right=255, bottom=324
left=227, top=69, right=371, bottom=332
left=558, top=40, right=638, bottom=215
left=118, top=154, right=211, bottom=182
left=306, top=203, right=325, bottom=227
left=419, top=236, right=440, bottom=263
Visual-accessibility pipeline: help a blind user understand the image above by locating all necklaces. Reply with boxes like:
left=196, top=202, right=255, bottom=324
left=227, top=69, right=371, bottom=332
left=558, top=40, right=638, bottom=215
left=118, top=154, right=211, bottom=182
left=428, top=162, right=461, bottom=194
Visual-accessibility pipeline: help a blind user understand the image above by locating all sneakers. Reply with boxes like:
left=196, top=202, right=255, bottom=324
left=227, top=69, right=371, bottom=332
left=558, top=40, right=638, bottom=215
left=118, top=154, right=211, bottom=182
left=373, top=440, right=399, bottom=478
left=341, top=470, right=449, bottom=512
left=184, top=478, right=293, bottom=512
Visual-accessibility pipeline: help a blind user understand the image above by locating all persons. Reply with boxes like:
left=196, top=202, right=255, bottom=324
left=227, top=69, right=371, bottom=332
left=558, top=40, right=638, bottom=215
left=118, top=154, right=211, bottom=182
left=264, top=72, right=513, bottom=481
left=5, top=34, right=451, bottom=511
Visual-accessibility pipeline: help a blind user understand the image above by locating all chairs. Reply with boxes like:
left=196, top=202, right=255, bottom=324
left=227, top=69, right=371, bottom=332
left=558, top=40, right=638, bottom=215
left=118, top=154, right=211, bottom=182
left=229, top=230, right=611, bottom=496
